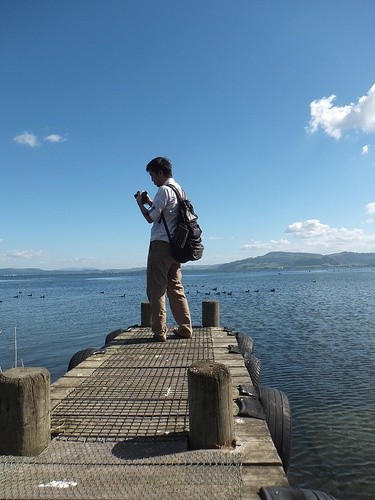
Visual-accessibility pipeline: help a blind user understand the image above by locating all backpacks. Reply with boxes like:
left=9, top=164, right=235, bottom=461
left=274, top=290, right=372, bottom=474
left=170, top=197, right=204, bottom=263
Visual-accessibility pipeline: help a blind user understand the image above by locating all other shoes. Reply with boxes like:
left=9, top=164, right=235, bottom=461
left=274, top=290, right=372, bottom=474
left=154, top=333, right=166, bottom=342
left=173, top=329, right=189, bottom=338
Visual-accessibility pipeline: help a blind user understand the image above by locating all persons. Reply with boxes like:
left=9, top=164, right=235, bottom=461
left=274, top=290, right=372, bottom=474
left=136, top=157, right=193, bottom=340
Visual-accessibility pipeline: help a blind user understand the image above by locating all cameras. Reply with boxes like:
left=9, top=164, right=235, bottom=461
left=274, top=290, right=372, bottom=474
left=134, top=191, right=148, bottom=204
